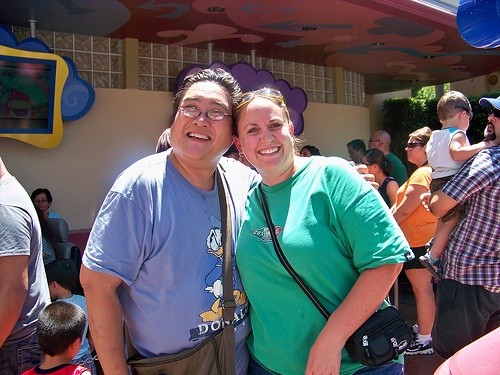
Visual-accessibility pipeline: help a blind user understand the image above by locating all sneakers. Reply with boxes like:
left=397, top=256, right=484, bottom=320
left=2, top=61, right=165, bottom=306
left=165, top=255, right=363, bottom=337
left=410, top=327, right=418, bottom=342
left=403, top=338, right=434, bottom=355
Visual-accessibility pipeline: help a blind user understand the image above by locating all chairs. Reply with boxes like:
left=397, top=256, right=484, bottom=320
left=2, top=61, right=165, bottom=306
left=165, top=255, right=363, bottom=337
left=47, top=217, right=82, bottom=272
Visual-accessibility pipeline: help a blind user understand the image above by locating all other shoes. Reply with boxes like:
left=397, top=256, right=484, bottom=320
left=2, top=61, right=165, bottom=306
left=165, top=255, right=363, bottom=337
left=419, top=250, right=442, bottom=281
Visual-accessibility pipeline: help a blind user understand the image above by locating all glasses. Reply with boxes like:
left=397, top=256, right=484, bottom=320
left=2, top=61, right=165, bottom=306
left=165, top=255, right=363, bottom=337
left=369, top=138, right=381, bottom=143
left=405, top=142, right=426, bottom=149
left=235, top=88, right=290, bottom=124
left=455, top=106, right=473, bottom=121
left=33, top=197, right=48, bottom=203
left=362, top=163, right=373, bottom=167
left=177, top=104, right=234, bottom=121
left=489, top=108, right=500, bottom=118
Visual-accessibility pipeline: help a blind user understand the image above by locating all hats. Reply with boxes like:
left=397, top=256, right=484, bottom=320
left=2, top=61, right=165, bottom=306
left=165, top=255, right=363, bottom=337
left=479, top=95, right=500, bottom=110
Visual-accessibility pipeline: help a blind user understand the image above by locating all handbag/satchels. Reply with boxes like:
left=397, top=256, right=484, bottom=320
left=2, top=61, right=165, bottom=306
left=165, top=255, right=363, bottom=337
left=126, top=324, right=236, bottom=375
left=344, top=305, right=412, bottom=367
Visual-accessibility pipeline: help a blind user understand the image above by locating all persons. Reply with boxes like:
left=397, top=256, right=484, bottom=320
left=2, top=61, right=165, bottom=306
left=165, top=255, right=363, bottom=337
left=156, top=128, right=172, bottom=153
left=80, top=67, right=379, bottom=375
left=300, top=92, right=500, bottom=375
left=232, top=87, right=415, bottom=375
left=0, top=155, right=103, bottom=375
